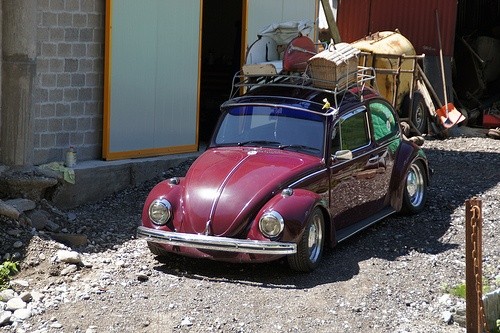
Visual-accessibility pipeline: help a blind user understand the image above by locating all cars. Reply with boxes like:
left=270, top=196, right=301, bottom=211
left=136, top=68, right=431, bottom=274
